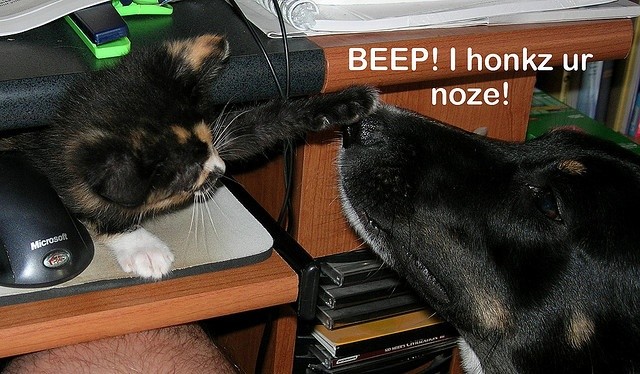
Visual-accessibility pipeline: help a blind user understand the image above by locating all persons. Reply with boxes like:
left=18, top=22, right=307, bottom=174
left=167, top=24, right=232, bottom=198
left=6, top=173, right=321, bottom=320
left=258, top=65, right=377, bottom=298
left=3, top=323, right=241, bottom=374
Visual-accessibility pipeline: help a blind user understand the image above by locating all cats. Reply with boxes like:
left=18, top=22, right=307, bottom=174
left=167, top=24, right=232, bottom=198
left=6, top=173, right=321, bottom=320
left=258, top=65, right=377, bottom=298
left=0, top=31, right=382, bottom=281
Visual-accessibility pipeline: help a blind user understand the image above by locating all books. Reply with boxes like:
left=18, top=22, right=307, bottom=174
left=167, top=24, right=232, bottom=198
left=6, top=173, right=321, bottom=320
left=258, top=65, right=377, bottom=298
left=258, top=1, right=640, bottom=39
left=526, top=63, right=640, bottom=150
left=296, top=260, right=460, bottom=374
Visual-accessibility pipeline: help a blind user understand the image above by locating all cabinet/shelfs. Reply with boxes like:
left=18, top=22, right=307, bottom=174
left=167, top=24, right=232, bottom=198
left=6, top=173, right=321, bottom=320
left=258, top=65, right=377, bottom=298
left=203, top=19, right=634, bottom=373
left=0, top=1, right=326, bottom=374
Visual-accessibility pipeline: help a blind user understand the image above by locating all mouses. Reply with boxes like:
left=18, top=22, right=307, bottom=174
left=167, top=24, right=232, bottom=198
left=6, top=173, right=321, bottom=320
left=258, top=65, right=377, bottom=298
left=0, top=149, right=94, bottom=289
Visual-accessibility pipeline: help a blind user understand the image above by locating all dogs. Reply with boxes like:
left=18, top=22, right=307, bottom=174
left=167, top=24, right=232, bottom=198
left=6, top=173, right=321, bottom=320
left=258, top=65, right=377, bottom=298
left=333, top=102, right=640, bottom=374
